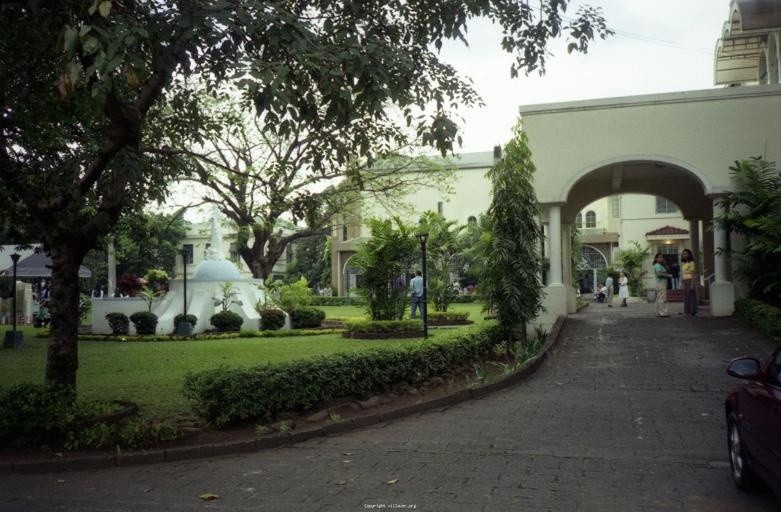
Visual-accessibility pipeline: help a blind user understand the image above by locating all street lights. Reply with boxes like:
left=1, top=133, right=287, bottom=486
left=8, top=251, right=24, bottom=347
left=414, top=230, right=431, bottom=338
left=178, top=247, right=192, bottom=339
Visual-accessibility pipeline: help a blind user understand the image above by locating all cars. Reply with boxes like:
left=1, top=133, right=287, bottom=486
left=723, top=349, right=781, bottom=510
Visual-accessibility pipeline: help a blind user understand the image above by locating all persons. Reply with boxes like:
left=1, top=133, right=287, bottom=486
left=605, top=274, right=615, bottom=307
left=582, top=274, right=592, bottom=292
left=597, top=283, right=608, bottom=303
left=653, top=253, right=674, bottom=317
left=90, top=282, right=104, bottom=298
left=680, top=248, right=699, bottom=315
left=618, top=272, right=629, bottom=307
left=410, top=270, right=427, bottom=321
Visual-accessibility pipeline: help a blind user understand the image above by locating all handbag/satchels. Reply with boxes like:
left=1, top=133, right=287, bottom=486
left=691, top=279, right=696, bottom=290
